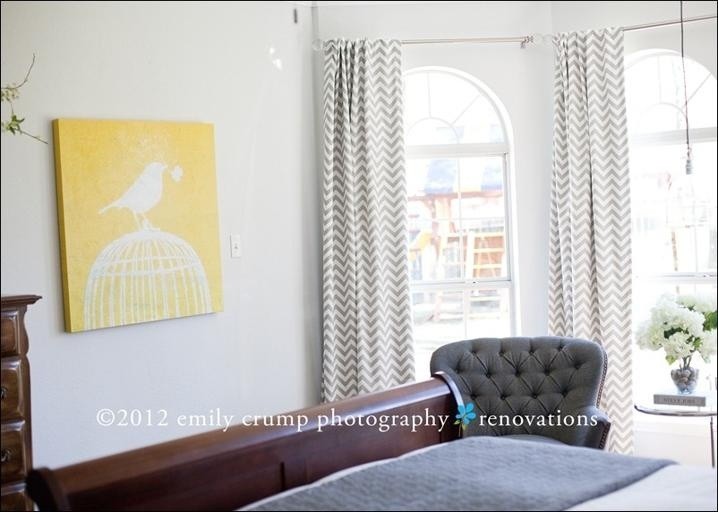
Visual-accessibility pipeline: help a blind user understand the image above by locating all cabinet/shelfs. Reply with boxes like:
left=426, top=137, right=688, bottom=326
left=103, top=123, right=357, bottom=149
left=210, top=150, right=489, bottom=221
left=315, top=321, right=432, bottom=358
left=1, top=294, right=44, bottom=510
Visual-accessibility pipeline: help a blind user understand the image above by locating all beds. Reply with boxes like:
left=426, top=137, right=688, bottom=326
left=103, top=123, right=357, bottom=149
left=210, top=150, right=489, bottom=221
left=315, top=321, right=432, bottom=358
left=23, top=371, right=718, bottom=511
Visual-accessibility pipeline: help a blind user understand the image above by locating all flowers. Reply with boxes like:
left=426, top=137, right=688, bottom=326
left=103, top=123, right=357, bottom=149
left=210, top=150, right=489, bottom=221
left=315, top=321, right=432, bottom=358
left=635, top=291, right=717, bottom=367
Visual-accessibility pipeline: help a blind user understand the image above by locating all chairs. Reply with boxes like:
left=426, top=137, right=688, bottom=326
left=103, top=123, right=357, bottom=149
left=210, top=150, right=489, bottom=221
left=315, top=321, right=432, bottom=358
left=431, top=337, right=611, bottom=450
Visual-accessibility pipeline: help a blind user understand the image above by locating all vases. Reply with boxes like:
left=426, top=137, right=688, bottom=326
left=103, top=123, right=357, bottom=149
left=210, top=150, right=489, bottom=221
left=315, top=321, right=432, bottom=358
left=671, top=367, right=699, bottom=395
